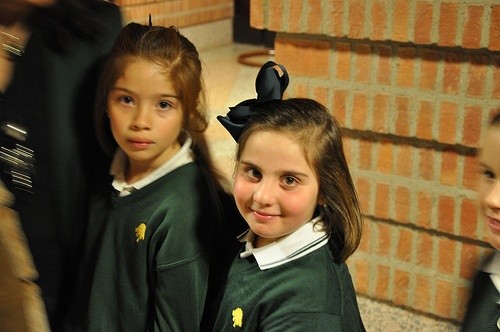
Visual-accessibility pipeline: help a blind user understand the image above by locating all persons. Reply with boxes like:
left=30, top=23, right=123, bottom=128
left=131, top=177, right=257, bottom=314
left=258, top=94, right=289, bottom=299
left=0, top=0, right=123, bottom=329
left=457, top=109, right=500, bottom=332
left=207, top=98, right=371, bottom=332
left=60, top=19, right=243, bottom=330
